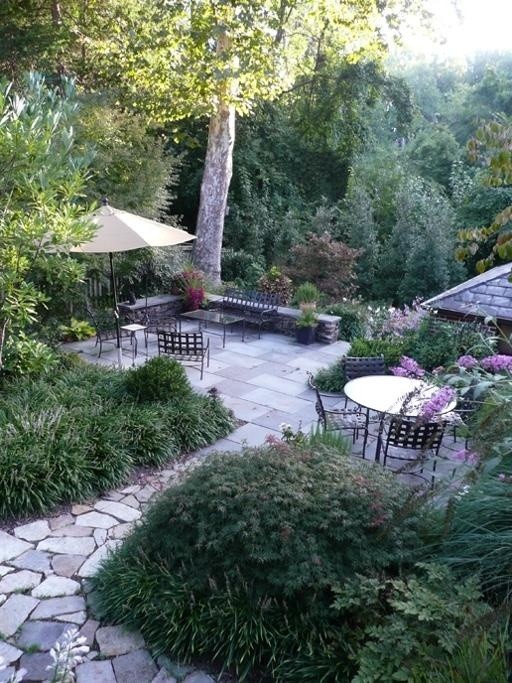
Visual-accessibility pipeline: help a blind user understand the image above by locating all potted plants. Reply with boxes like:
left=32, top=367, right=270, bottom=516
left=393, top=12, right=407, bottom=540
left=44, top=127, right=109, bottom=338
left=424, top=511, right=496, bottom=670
left=290, top=281, right=321, bottom=345
left=58, top=317, right=96, bottom=342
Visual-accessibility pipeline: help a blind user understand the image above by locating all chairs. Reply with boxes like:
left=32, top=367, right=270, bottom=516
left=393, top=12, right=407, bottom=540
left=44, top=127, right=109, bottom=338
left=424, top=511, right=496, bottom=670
left=85, top=307, right=136, bottom=357
left=157, top=330, right=210, bottom=380
left=306, top=352, right=482, bottom=491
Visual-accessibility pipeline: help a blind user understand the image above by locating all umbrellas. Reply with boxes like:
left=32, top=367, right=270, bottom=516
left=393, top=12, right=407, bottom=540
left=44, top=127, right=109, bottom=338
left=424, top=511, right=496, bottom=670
left=32, top=198, right=197, bottom=367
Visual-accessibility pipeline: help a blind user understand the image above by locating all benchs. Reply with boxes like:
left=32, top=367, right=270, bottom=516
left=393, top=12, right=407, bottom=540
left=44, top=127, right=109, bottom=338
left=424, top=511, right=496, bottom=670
left=205, top=289, right=281, bottom=340
